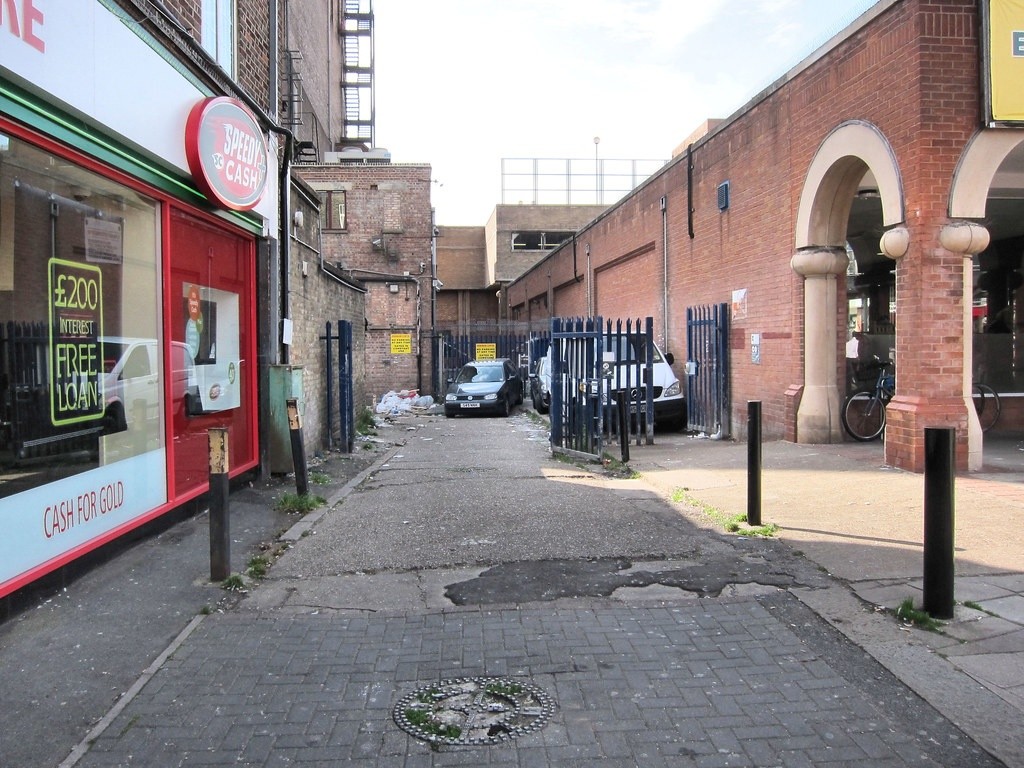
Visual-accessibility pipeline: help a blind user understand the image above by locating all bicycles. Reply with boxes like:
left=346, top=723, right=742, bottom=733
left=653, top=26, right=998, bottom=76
left=840, top=357, right=1003, bottom=443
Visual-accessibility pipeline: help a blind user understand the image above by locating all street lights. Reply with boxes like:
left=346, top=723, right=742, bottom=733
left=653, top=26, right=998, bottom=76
left=593, top=135, right=601, bottom=204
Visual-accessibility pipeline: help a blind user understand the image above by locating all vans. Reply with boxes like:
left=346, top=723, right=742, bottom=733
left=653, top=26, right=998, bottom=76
left=70, top=333, right=198, bottom=450
left=545, top=329, right=687, bottom=433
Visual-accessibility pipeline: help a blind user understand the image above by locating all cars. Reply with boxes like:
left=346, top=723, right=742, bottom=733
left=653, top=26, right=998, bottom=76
left=528, top=356, right=548, bottom=414
left=443, top=357, right=523, bottom=417
